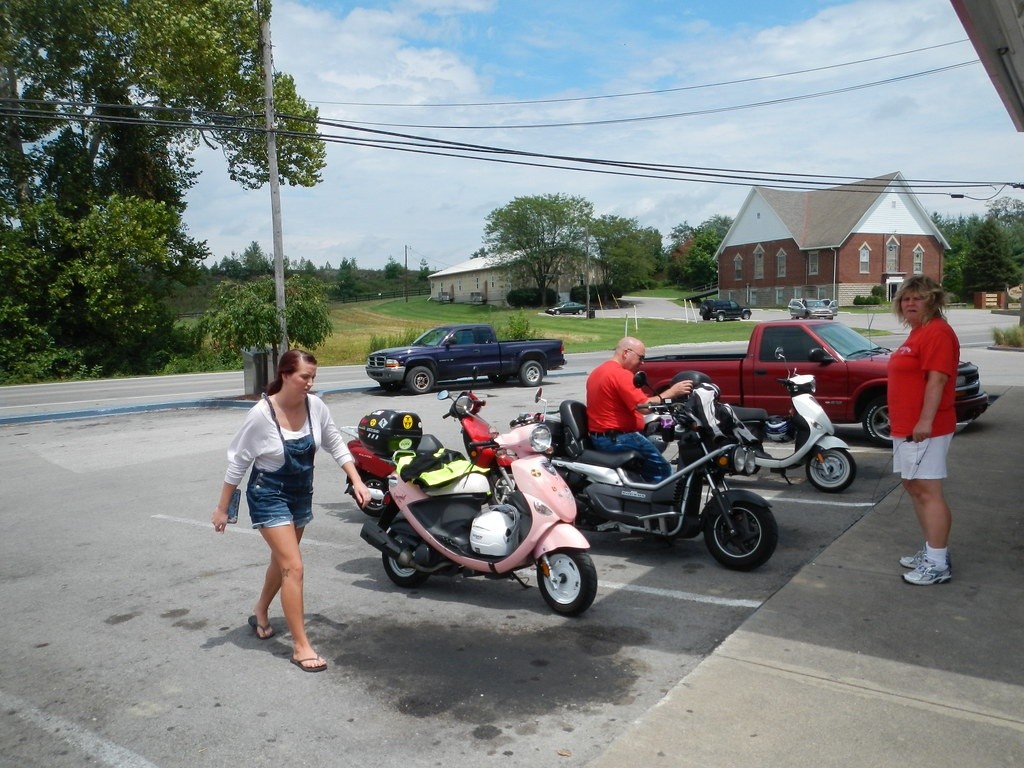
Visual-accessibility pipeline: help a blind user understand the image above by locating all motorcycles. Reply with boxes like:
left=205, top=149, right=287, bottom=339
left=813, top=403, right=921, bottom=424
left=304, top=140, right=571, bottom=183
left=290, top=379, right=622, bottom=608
left=346, top=367, right=521, bottom=518
left=359, top=412, right=599, bottom=618
left=668, top=345, right=857, bottom=494
left=542, top=372, right=780, bottom=571
left=508, top=386, right=675, bottom=459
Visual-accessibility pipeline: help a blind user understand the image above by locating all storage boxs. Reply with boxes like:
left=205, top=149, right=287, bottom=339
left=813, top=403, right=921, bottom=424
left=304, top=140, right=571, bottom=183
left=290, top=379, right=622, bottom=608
left=358, top=408, right=423, bottom=456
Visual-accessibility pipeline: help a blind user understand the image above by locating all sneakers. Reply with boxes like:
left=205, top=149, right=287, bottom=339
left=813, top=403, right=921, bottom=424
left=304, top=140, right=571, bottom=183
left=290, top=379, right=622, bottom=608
left=899, top=545, right=953, bottom=569
left=900, top=555, right=952, bottom=586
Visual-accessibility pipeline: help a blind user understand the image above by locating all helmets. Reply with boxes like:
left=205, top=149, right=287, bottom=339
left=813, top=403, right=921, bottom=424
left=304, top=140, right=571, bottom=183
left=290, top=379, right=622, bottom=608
left=671, top=370, right=712, bottom=404
left=766, top=415, right=796, bottom=444
left=470, top=504, right=522, bottom=557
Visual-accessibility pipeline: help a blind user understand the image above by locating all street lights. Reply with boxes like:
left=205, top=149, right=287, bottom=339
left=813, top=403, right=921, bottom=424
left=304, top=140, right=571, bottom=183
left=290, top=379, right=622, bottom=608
left=585, top=223, right=590, bottom=319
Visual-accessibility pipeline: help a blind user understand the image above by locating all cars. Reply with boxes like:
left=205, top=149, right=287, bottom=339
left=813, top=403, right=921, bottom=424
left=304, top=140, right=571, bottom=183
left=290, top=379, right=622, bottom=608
left=545, top=301, right=587, bottom=315
left=790, top=298, right=839, bottom=320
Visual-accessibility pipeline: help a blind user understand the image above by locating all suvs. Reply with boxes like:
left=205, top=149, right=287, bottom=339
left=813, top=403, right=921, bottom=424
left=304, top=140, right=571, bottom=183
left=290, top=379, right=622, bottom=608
left=699, top=299, right=753, bottom=322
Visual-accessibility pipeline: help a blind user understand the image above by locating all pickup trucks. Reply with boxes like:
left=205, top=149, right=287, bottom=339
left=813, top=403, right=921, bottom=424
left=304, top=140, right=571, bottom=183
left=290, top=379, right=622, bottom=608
left=628, top=320, right=991, bottom=450
left=365, top=324, right=566, bottom=395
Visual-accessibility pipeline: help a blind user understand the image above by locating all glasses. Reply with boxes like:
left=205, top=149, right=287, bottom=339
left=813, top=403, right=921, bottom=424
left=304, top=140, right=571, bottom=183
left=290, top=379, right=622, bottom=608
left=628, top=349, right=645, bottom=361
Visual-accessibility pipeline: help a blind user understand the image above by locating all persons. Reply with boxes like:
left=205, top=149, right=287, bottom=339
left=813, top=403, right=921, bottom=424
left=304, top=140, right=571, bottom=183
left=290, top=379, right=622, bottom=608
left=209, top=348, right=374, bottom=673
left=585, top=337, right=693, bottom=480
left=888, top=276, right=959, bottom=585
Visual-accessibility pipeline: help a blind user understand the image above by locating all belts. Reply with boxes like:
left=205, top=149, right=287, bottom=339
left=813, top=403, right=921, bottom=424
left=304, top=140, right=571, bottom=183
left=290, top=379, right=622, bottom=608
left=587, top=429, right=633, bottom=437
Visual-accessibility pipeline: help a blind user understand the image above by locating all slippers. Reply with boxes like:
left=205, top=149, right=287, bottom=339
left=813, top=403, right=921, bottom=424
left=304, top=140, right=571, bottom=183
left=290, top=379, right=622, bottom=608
left=248, top=615, right=275, bottom=639
left=290, top=653, right=327, bottom=672
